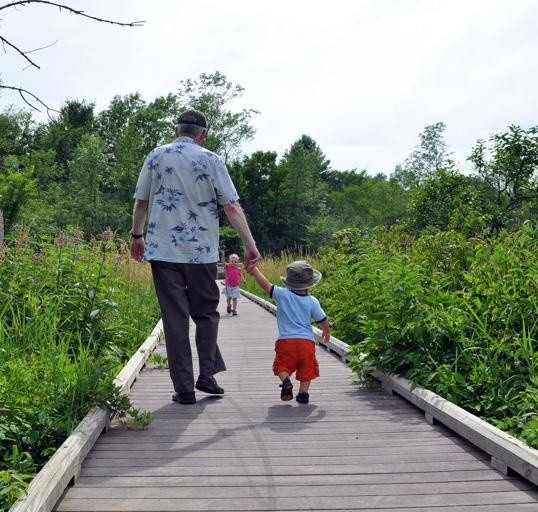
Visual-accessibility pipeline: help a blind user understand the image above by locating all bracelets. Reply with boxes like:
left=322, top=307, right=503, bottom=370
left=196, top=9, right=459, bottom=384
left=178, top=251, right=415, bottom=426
left=131, top=233, right=142, bottom=239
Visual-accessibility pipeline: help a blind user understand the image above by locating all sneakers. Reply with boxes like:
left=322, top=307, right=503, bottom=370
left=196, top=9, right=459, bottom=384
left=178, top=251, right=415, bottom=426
left=171, top=392, right=197, bottom=404
left=296, top=392, right=309, bottom=403
left=227, top=305, right=231, bottom=313
left=233, top=310, right=237, bottom=315
left=196, top=375, right=224, bottom=394
left=281, top=377, right=293, bottom=401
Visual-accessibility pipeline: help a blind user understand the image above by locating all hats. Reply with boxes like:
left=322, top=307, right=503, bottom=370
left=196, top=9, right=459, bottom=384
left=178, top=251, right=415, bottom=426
left=279, top=261, right=323, bottom=290
left=178, top=109, right=208, bottom=129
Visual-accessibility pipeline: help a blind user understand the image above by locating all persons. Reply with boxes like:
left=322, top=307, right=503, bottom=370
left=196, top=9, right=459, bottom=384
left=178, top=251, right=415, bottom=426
left=242, top=259, right=330, bottom=404
left=128, top=111, right=262, bottom=405
left=218, top=248, right=247, bottom=316
